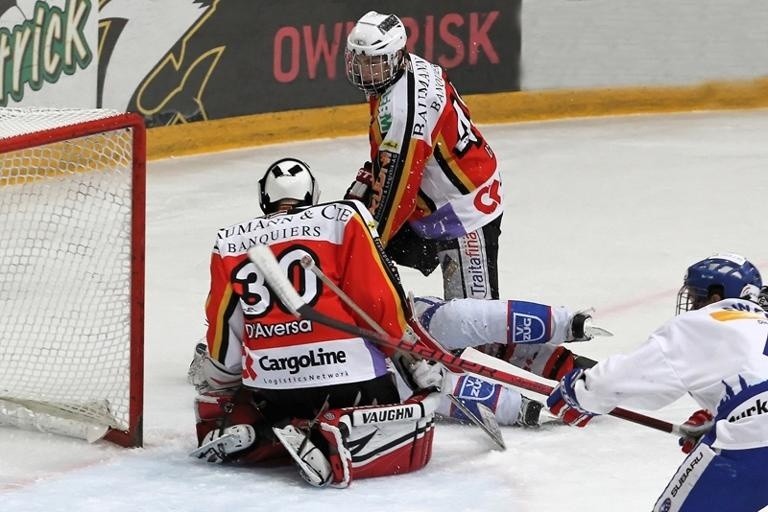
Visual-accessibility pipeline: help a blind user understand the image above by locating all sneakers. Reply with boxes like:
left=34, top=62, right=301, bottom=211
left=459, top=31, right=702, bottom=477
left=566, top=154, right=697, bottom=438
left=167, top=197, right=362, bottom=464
left=515, top=394, right=543, bottom=430
left=201, top=422, right=256, bottom=464
left=272, top=424, right=333, bottom=487
left=564, top=311, right=594, bottom=344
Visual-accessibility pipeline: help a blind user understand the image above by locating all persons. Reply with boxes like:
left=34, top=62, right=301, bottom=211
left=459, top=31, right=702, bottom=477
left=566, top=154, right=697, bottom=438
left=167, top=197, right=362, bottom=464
left=345, top=10, right=503, bottom=302
left=189, top=157, right=442, bottom=490
left=546, top=252, right=768, bottom=511
left=404, top=294, right=614, bottom=429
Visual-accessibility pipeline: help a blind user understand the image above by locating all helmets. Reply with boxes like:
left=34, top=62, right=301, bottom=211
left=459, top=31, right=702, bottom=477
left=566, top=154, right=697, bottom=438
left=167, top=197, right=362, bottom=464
left=676, top=252, right=762, bottom=313
left=344, top=10, right=408, bottom=95
left=258, top=157, right=321, bottom=215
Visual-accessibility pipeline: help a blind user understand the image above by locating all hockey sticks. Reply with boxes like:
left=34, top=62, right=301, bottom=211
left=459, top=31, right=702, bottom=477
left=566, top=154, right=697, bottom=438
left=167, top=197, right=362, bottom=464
left=300, top=255, right=508, bottom=452
left=248, top=245, right=703, bottom=441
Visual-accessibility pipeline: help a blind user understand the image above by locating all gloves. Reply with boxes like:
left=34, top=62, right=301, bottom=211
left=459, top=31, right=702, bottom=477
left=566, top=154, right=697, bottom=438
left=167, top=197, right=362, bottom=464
left=677, top=409, right=717, bottom=453
left=547, top=368, right=602, bottom=428
